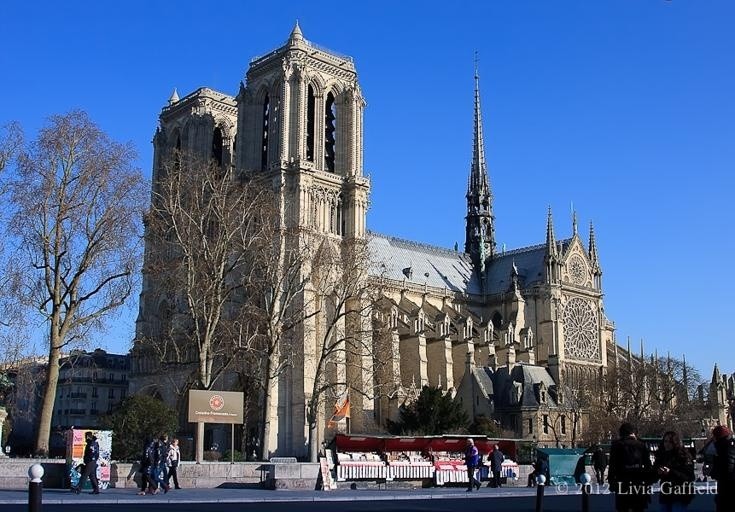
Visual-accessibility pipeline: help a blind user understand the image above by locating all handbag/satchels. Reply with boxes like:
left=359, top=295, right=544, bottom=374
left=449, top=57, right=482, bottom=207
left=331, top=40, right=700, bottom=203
left=171, top=459, right=178, bottom=469
left=628, top=463, right=659, bottom=491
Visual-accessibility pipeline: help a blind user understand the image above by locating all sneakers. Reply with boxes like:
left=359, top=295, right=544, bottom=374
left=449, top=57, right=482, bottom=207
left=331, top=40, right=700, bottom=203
left=133, top=484, right=182, bottom=496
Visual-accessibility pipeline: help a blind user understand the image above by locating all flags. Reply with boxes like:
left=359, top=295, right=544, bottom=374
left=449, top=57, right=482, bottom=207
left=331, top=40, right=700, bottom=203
left=326, top=387, right=351, bottom=429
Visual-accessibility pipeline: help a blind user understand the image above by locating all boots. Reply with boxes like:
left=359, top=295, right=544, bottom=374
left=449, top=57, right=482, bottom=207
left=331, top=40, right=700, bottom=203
left=69, top=483, right=99, bottom=495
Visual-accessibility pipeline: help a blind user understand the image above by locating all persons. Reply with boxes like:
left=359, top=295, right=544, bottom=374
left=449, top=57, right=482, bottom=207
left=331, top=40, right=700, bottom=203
left=69, top=432, right=101, bottom=496
left=487, top=443, right=505, bottom=488
left=698, top=424, right=735, bottom=512
left=135, top=431, right=183, bottom=497
left=590, top=447, right=609, bottom=488
left=606, top=423, right=657, bottom=512
left=526, top=456, right=550, bottom=488
left=653, top=429, right=697, bottom=512
left=461, top=438, right=482, bottom=493
